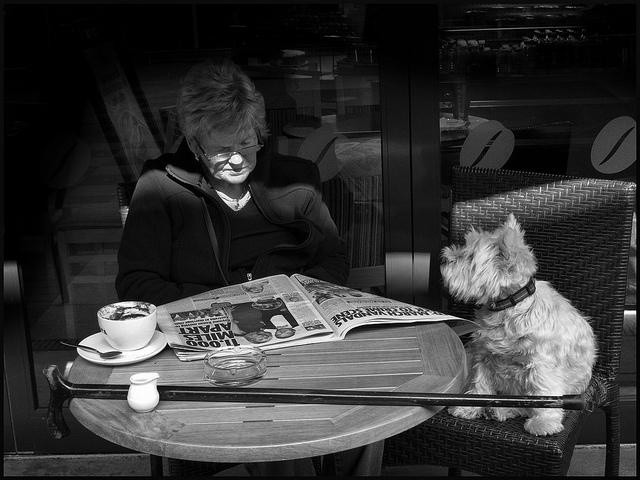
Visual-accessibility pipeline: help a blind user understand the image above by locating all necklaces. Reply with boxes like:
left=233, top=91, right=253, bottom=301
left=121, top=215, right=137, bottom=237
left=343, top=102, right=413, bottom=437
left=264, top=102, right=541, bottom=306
left=211, top=186, right=249, bottom=209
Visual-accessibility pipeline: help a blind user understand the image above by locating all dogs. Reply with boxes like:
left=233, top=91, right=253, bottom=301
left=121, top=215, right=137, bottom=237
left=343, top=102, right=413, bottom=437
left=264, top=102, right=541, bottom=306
left=438, top=212, right=602, bottom=439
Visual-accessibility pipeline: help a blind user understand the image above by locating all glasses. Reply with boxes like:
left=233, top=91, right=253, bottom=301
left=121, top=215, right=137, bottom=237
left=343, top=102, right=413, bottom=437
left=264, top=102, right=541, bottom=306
left=193, top=126, right=265, bottom=161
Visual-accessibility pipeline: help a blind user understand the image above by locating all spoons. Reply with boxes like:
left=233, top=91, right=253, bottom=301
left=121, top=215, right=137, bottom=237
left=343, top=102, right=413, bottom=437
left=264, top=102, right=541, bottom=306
left=58, top=340, right=122, bottom=359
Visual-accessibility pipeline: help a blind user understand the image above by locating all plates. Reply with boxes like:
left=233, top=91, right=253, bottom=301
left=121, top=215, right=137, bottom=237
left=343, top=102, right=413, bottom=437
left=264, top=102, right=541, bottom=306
left=77, top=330, right=168, bottom=366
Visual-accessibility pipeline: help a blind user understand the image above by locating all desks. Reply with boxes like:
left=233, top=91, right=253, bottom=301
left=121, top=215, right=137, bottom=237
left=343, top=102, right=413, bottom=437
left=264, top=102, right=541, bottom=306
left=68, top=323, right=466, bottom=478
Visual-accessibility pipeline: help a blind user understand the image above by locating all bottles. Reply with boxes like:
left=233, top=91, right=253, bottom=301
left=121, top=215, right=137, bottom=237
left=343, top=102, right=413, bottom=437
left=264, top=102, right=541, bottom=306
left=127, top=371, right=159, bottom=414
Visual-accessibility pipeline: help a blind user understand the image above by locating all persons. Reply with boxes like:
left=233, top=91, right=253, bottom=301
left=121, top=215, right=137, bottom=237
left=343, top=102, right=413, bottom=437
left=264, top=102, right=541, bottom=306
left=114, top=57, right=349, bottom=306
left=231, top=299, right=298, bottom=344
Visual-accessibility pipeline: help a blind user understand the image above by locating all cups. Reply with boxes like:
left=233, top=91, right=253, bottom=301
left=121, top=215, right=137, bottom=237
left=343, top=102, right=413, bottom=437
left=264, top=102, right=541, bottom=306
left=97, top=300, right=158, bottom=351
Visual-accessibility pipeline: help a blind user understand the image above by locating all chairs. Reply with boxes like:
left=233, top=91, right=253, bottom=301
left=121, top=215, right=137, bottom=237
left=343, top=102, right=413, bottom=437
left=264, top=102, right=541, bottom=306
left=384, top=163, right=637, bottom=476
left=117, top=181, right=346, bottom=477
